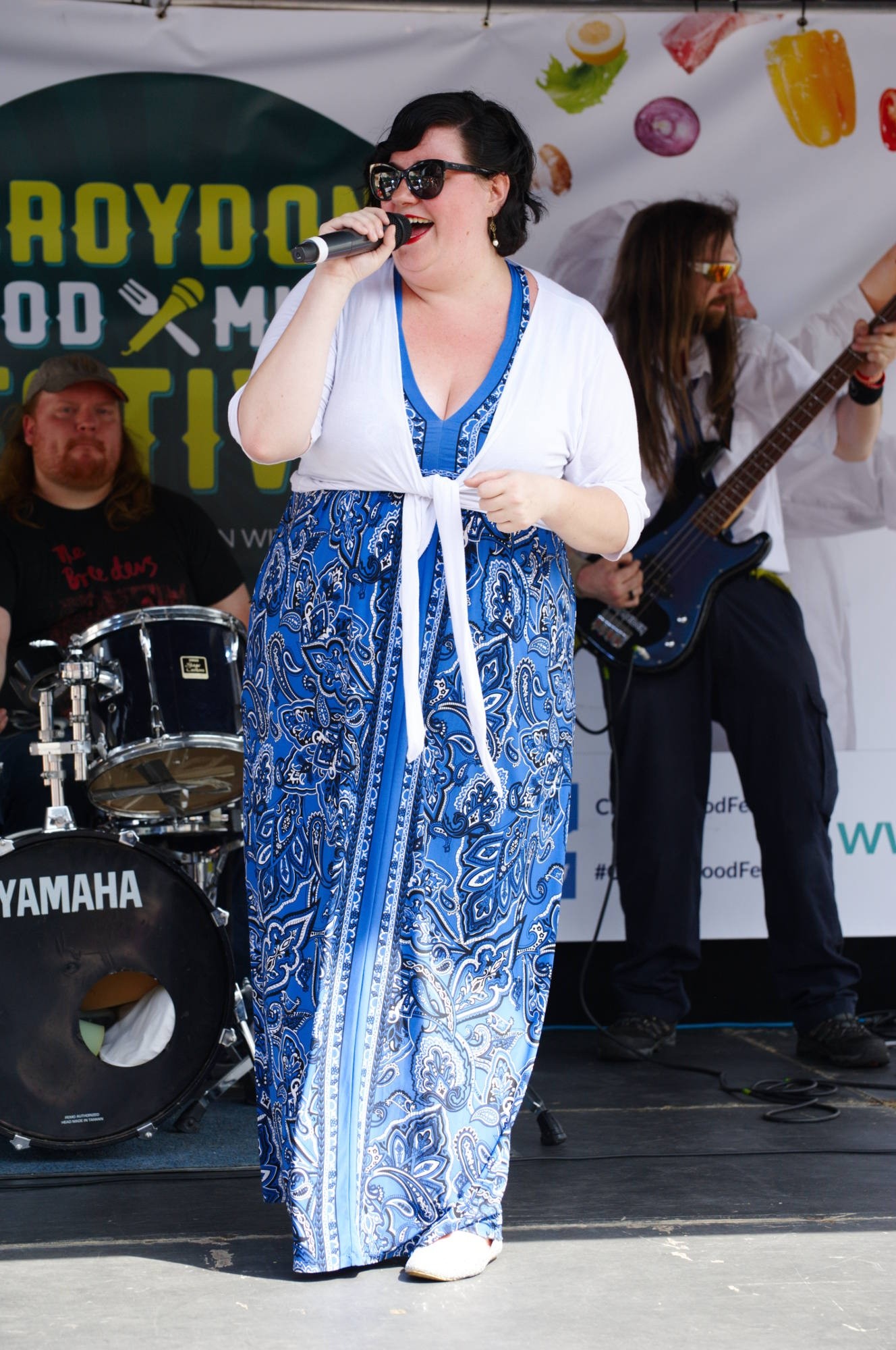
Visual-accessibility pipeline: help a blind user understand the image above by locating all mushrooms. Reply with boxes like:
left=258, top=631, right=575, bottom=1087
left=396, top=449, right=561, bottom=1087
left=530, top=145, right=574, bottom=196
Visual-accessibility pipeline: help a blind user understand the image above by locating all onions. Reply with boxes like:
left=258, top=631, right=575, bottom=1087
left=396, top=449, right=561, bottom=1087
left=637, top=97, right=700, bottom=158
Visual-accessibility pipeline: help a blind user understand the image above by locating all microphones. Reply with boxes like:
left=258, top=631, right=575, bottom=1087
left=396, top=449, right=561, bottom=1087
left=291, top=211, right=412, bottom=265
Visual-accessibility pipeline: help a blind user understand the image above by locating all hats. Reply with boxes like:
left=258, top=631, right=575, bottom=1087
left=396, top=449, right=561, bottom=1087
left=23, top=354, right=129, bottom=411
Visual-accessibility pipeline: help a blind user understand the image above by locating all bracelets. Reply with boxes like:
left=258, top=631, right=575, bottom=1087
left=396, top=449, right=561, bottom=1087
left=848, top=369, right=885, bottom=406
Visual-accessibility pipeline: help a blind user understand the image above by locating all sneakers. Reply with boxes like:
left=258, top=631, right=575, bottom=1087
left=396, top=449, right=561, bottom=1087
left=406, top=1225, right=503, bottom=1282
left=597, top=1011, right=677, bottom=1061
left=793, top=1010, right=888, bottom=1067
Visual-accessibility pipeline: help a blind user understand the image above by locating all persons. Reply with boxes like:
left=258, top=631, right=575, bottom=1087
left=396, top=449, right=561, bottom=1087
left=0, top=361, right=258, bottom=733
left=569, top=196, right=896, bottom=1072
left=228, top=91, right=648, bottom=1284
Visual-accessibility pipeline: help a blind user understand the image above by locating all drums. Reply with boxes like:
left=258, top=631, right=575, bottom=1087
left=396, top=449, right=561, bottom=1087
left=67, top=605, right=245, bottom=852
left=0, top=826, right=236, bottom=1152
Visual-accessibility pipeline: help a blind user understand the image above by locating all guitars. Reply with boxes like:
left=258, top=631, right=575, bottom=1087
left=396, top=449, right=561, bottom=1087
left=576, top=292, right=896, bottom=673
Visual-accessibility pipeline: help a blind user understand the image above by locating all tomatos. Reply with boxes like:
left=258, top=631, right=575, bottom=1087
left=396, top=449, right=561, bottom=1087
left=879, top=88, right=896, bottom=152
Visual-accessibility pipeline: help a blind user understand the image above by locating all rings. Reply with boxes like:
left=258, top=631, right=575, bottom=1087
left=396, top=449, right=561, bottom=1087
left=629, top=590, right=634, bottom=600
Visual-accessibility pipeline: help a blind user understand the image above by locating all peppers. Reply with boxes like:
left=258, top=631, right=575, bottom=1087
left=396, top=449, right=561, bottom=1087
left=765, top=19, right=856, bottom=148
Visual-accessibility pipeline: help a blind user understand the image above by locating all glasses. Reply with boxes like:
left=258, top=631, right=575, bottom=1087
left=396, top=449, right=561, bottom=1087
left=369, top=159, right=496, bottom=201
left=684, top=262, right=736, bottom=284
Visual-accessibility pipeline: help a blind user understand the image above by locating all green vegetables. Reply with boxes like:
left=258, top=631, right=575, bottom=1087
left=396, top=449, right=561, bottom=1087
left=537, top=47, right=627, bottom=113
